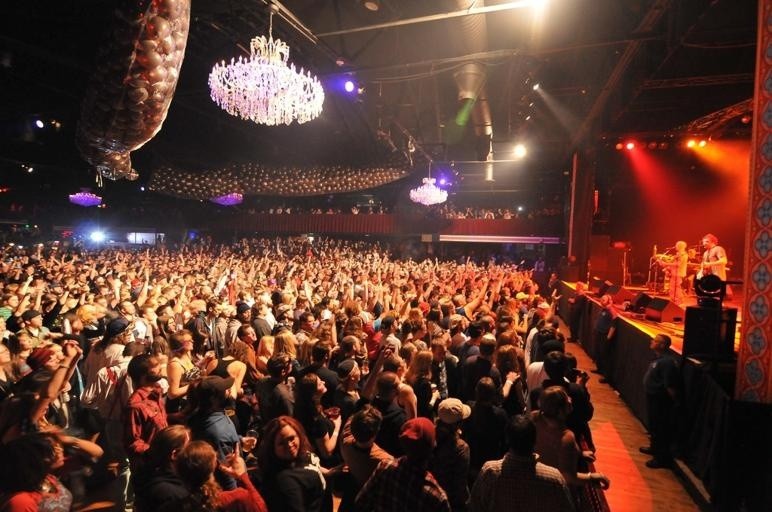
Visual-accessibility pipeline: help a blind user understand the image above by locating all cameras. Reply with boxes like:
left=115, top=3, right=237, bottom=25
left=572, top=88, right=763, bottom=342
left=577, top=369, right=590, bottom=379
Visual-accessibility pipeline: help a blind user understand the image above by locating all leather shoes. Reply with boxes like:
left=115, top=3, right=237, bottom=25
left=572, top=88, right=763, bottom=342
left=646, top=460, right=668, bottom=469
left=640, top=447, right=652, bottom=454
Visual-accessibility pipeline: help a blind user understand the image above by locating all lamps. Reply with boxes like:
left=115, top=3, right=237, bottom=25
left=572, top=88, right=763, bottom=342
left=409, top=161, right=449, bottom=207
left=206, top=3, right=326, bottom=128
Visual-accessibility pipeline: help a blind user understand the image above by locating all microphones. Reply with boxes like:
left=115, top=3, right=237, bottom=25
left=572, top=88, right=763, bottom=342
left=666, top=246, right=676, bottom=251
left=694, top=244, right=704, bottom=250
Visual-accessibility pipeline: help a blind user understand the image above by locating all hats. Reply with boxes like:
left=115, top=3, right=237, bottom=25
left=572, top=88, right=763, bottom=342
left=516, top=292, right=528, bottom=298
left=381, top=316, right=396, bottom=332
left=271, top=323, right=288, bottom=337
left=399, top=418, right=435, bottom=454
left=268, top=352, right=291, bottom=372
left=199, top=375, right=235, bottom=401
left=274, top=304, right=291, bottom=317
left=437, top=398, right=472, bottom=424
left=449, top=314, right=462, bottom=329
left=338, top=360, right=355, bottom=379
left=103, top=317, right=131, bottom=344
left=26, top=348, right=54, bottom=370
left=22, top=310, right=41, bottom=321
left=236, top=301, right=251, bottom=313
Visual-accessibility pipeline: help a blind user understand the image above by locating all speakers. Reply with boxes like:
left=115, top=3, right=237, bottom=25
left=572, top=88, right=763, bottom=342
left=681, top=305, right=737, bottom=361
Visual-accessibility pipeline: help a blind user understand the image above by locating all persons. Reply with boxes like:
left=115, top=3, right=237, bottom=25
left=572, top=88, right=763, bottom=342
left=659, top=240, right=689, bottom=305
left=687, top=249, right=699, bottom=264
left=696, top=233, right=729, bottom=283
left=638, top=334, right=681, bottom=468
left=0, top=199, right=609, bottom=512
left=590, top=294, right=618, bottom=384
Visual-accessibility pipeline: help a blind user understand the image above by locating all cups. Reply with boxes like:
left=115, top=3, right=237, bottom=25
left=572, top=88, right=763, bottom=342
left=323, top=407, right=341, bottom=420
left=242, top=429, right=259, bottom=453
left=214, top=445, right=234, bottom=466
